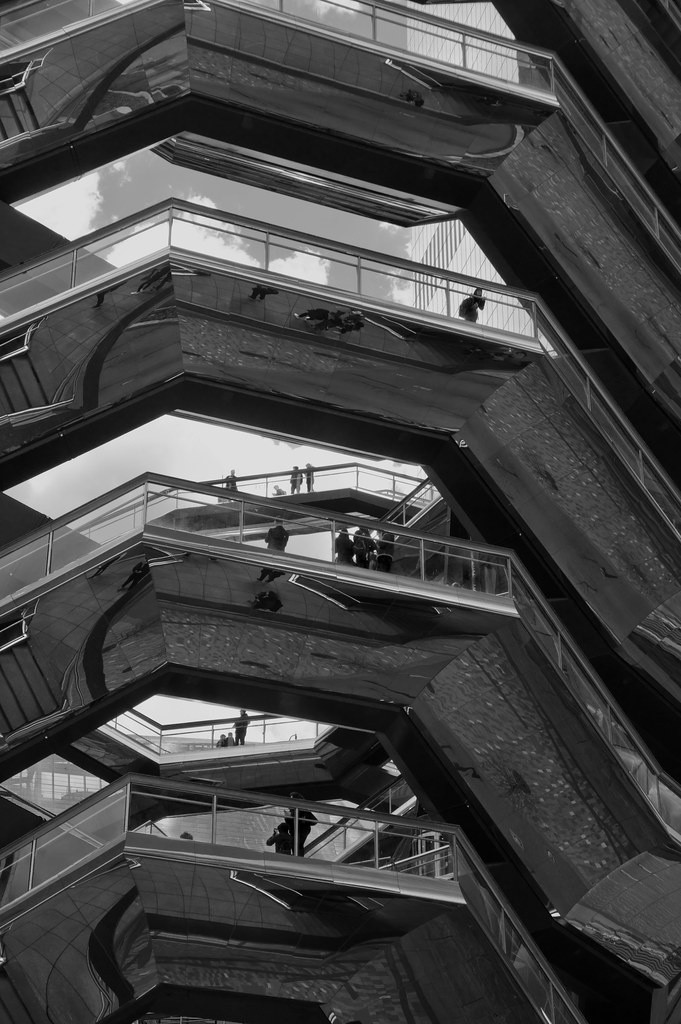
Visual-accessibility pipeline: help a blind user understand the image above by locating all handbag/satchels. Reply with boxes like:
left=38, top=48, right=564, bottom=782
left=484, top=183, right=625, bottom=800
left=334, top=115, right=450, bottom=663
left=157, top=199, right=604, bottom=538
left=305, top=812, right=318, bottom=826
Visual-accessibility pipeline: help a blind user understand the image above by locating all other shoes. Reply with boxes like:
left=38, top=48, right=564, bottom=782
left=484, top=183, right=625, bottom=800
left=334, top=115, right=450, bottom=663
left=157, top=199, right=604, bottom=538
left=257, top=578, right=262, bottom=581
left=293, top=313, right=300, bottom=319
left=306, top=317, right=310, bottom=320
left=140, top=289, right=143, bottom=292
left=131, top=292, right=139, bottom=295
left=265, top=580, right=270, bottom=582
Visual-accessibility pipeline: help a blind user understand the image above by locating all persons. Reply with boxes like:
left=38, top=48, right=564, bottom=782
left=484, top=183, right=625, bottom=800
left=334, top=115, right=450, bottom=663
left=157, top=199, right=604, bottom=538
left=256, top=568, right=285, bottom=583
left=294, top=307, right=365, bottom=335
left=274, top=485, right=287, bottom=496
left=332, top=524, right=394, bottom=573
left=92, top=292, right=107, bottom=309
left=398, top=89, right=424, bottom=107
left=216, top=733, right=234, bottom=748
left=464, top=288, right=486, bottom=322
left=234, top=708, right=250, bottom=746
left=290, top=466, right=303, bottom=493
left=117, top=560, right=149, bottom=593
left=266, top=792, right=318, bottom=858
left=131, top=264, right=172, bottom=295
left=225, top=470, right=238, bottom=491
left=306, top=462, right=316, bottom=491
left=250, top=283, right=279, bottom=302
left=249, top=591, right=283, bottom=612
left=264, top=517, right=289, bottom=552
